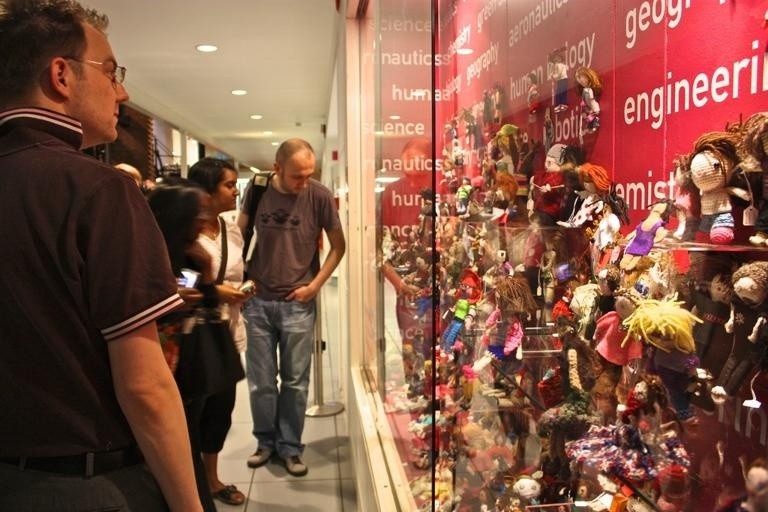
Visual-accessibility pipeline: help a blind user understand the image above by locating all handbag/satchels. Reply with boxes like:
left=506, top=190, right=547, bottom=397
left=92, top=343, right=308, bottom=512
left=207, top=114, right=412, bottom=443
left=176, top=318, right=245, bottom=401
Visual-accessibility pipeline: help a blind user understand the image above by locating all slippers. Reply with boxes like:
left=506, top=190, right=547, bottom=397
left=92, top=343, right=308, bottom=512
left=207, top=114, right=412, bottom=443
left=210, top=485, right=244, bottom=506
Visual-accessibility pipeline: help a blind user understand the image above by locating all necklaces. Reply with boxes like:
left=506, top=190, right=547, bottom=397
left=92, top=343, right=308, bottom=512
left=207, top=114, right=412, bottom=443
left=204, top=220, right=219, bottom=240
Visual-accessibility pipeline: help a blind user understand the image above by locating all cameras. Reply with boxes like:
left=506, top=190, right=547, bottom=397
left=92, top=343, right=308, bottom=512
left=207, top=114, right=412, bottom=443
left=239, top=280, right=255, bottom=294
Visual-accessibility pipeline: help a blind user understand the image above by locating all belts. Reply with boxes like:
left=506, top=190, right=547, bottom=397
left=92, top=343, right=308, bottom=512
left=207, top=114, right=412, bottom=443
left=5, top=446, right=140, bottom=477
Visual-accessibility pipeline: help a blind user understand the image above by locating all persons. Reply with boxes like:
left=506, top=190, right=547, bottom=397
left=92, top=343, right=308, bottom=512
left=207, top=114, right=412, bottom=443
left=233, top=137, right=346, bottom=478
left=112, top=162, right=156, bottom=192
left=188, top=157, right=256, bottom=506
left=147, top=178, right=220, bottom=512
left=0, top=0, right=204, bottom=512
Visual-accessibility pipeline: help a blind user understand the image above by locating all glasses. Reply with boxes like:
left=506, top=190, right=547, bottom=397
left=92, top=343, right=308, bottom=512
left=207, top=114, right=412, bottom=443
left=61, top=54, right=126, bottom=83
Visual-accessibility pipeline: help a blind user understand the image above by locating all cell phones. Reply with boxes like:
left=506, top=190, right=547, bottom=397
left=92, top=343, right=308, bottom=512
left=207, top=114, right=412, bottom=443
left=177, top=268, right=201, bottom=288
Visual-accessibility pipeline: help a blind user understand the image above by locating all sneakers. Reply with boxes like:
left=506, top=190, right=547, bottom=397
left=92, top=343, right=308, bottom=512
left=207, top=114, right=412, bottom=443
left=246, top=447, right=275, bottom=468
left=281, top=453, right=306, bottom=475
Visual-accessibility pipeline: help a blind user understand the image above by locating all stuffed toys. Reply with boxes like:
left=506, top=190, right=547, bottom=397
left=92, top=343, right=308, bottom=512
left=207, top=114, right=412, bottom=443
left=380, top=44, right=768, bottom=512
left=383, top=43, right=768, bottom=512
